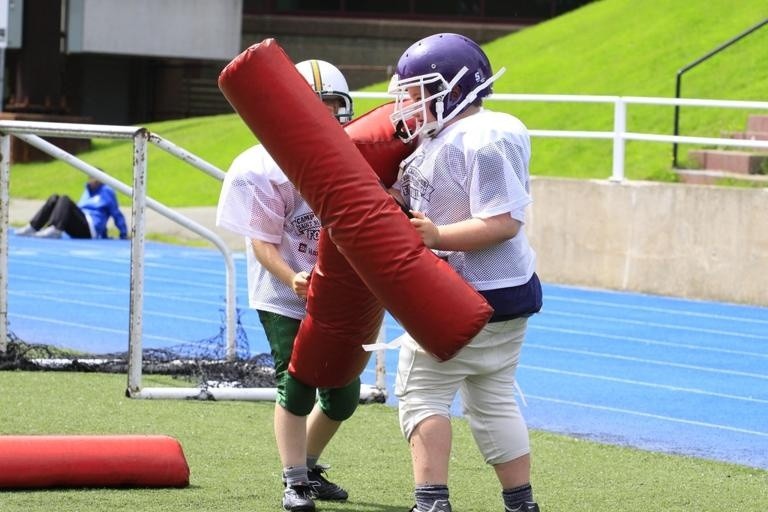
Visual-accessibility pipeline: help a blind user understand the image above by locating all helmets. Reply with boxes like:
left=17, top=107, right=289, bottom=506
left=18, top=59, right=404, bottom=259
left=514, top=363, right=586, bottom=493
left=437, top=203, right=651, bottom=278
left=293, top=58, right=353, bottom=124
left=386, top=33, right=494, bottom=145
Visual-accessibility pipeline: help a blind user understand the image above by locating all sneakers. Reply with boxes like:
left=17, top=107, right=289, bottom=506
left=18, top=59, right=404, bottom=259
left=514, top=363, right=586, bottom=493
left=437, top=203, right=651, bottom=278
left=32, top=225, right=62, bottom=238
left=505, top=501, right=539, bottom=512
left=14, top=224, right=37, bottom=236
left=409, top=500, right=451, bottom=512
left=282, top=463, right=348, bottom=501
left=282, top=479, right=315, bottom=512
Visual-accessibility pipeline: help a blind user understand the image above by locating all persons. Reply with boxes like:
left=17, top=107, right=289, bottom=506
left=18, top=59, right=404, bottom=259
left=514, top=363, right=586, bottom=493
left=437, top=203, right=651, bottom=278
left=214, top=57, right=361, bottom=510
left=385, top=32, right=544, bottom=511
left=10, top=165, right=129, bottom=237
